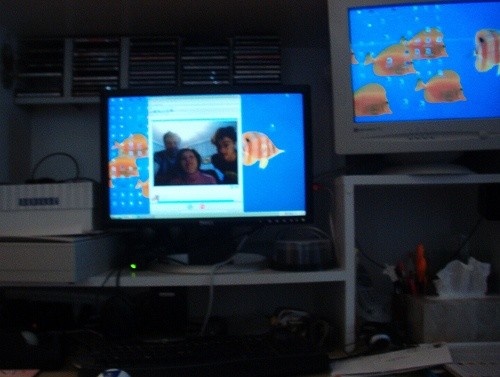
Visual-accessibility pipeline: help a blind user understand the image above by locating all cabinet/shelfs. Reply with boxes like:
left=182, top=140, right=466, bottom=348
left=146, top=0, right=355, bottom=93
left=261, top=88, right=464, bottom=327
left=81, top=172, right=500, bottom=354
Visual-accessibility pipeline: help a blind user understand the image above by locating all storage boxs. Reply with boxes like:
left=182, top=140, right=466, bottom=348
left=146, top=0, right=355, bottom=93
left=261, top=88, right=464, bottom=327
left=412, top=294, right=500, bottom=344
left=0, top=238, right=111, bottom=283
left=1, top=179, right=101, bottom=239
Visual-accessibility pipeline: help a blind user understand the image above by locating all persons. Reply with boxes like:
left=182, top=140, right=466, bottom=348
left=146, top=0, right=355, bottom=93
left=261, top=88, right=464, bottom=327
left=209, top=126, right=239, bottom=184
left=169, top=148, right=218, bottom=185
left=153, top=130, right=185, bottom=185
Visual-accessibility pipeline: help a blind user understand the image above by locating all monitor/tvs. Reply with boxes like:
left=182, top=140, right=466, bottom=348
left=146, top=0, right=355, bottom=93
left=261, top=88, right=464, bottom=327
left=327, top=0, right=497, bottom=156
left=100, top=84, right=317, bottom=230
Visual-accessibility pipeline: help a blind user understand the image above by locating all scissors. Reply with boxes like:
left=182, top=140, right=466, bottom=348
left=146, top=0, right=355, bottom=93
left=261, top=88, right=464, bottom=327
left=415, top=243, right=426, bottom=288
